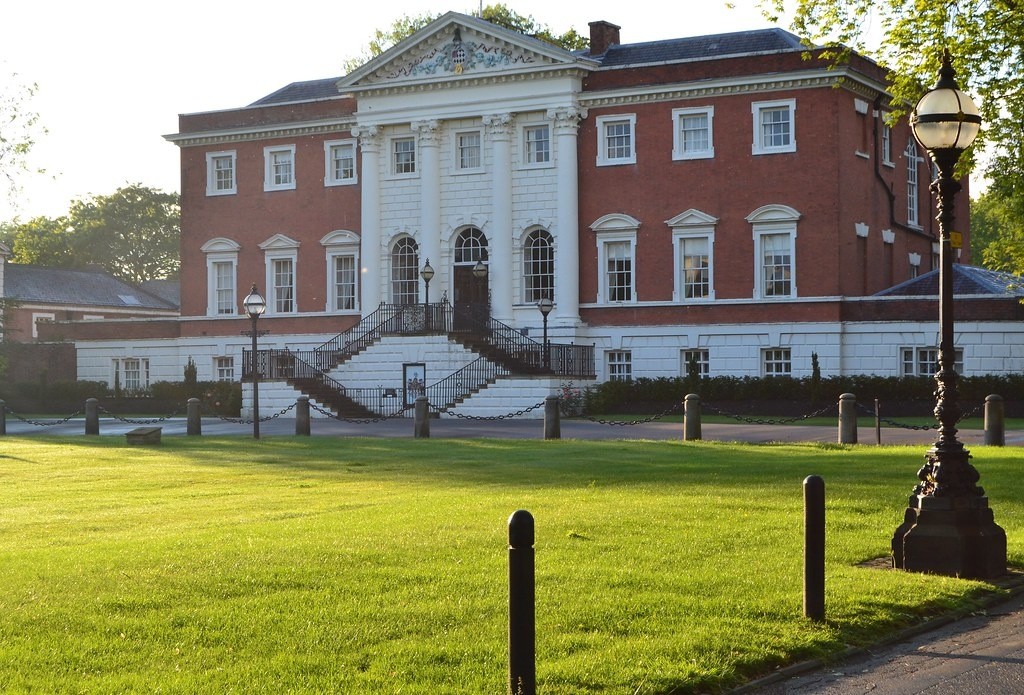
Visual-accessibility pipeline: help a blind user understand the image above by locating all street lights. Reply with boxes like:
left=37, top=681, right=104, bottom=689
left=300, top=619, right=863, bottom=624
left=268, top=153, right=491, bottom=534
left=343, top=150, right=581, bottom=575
left=880, top=48, right=1007, bottom=582
left=243, top=283, right=267, bottom=440
